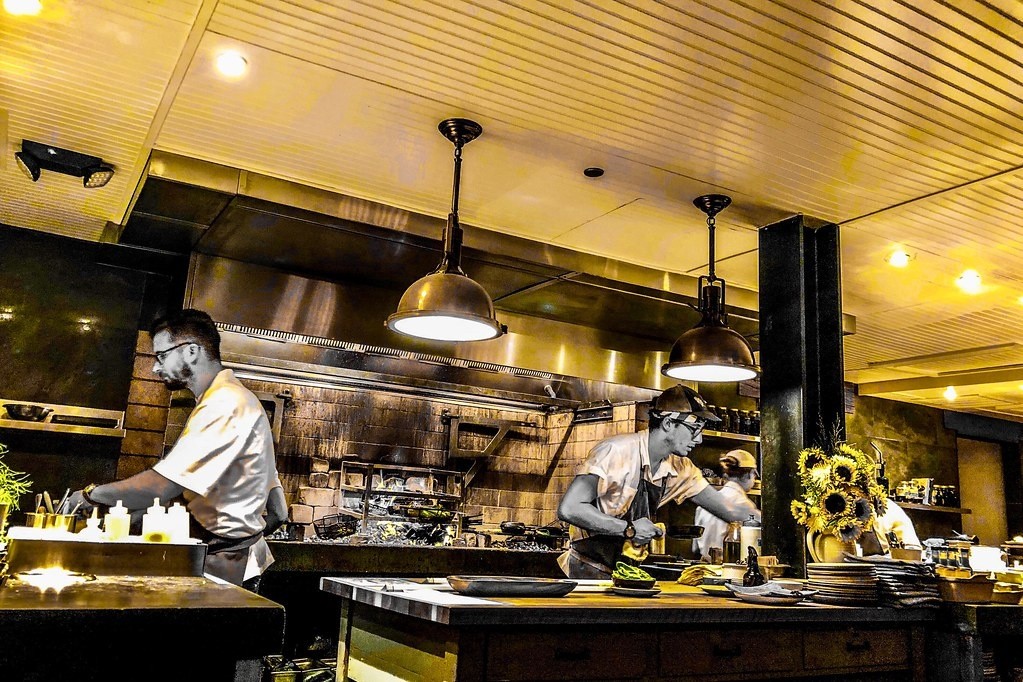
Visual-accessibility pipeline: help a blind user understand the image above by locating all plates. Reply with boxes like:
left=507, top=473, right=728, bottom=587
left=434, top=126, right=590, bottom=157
left=702, top=587, right=734, bottom=597
left=654, top=561, right=691, bottom=567
left=1005, top=541, right=1023, bottom=544
left=49, top=414, right=120, bottom=429
left=610, top=587, right=662, bottom=598
left=727, top=562, right=879, bottom=609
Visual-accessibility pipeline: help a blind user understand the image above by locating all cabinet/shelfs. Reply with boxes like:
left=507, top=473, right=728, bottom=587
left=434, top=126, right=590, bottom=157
left=702, top=429, right=765, bottom=497
left=338, top=460, right=467, bottom=543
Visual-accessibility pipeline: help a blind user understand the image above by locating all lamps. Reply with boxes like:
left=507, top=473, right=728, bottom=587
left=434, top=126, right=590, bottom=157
left=383, top=110, right=510, bottom=344
left=657, top=191, right=764, bottom=387
left=13, top=138, right=115, bottom=191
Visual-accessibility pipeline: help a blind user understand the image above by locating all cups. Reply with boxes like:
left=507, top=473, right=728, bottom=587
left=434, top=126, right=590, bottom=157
left=23, top=512, right=77, bottom=533
left=889, top=547, right=922, bottom=561
left=708, top=547, right=723, bottom=565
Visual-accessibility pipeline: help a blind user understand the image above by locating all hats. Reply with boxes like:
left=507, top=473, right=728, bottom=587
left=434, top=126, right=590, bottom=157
left=655, top=384, right=723, bottom=422
left=727, top=449, right=760, bottom=479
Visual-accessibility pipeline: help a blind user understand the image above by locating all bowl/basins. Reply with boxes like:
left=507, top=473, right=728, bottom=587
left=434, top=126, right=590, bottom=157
left=665, top=524, right=705, bottom=539
left=447, top=575, right=579, bottom=597
left=759, top=563, right=791, bottom=578
left=2, top=404, right=54, bottom=422
left=767, top=579, right=803, bottom=590
left=611, top=577, right=656, bottom=590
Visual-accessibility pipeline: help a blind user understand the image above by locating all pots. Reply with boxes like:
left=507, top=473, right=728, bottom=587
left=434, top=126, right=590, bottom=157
left=500, top=521, right=533, bottom=534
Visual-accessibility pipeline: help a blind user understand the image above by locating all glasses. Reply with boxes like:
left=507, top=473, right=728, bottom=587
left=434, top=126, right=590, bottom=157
left=666, top=418, right=707, bottom=437
left=154, top=342, right=201, bottom=365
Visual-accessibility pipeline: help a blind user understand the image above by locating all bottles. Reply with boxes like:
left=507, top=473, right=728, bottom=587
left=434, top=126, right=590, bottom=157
left=104, top=499, right=132, bottom=542
left=142, top=497, right=168, bottom=542
left=742, top=546, right=764, bottom=587
left=878, top=463, right=959, bottom=507
left=930, top=546, right=973, bottom=578
left=723, top=514, right=761, bottom=563
left=703, top=404, right=761, bottom=436
left=74, top=506, right=103, bottom=541
left=166, top=502, right=190, bottom=544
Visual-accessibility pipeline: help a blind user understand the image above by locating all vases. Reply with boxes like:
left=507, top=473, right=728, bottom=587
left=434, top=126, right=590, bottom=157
left=807, top=528, right=857, bottom=562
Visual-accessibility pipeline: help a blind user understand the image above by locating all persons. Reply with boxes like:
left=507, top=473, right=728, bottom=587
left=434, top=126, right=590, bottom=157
left=692, top=449, right=761, bottom=564
left=558, top=384, right=761, bottom=580
left=58, top=309, right=288, bottom=682
left=854, top=453, right=922, bottom=557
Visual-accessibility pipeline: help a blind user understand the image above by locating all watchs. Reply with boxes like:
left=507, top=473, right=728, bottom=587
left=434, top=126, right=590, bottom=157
left=82, top=483, right=100, bottom=505
left=623, top=520, right=636, bottom=539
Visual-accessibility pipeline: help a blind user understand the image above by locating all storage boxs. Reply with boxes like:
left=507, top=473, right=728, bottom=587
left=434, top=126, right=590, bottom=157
left=938, top=571, right=998, bottom=604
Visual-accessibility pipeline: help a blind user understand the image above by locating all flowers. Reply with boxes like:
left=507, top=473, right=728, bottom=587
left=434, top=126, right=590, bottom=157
left=790, top=440, right=890, bottom=542
